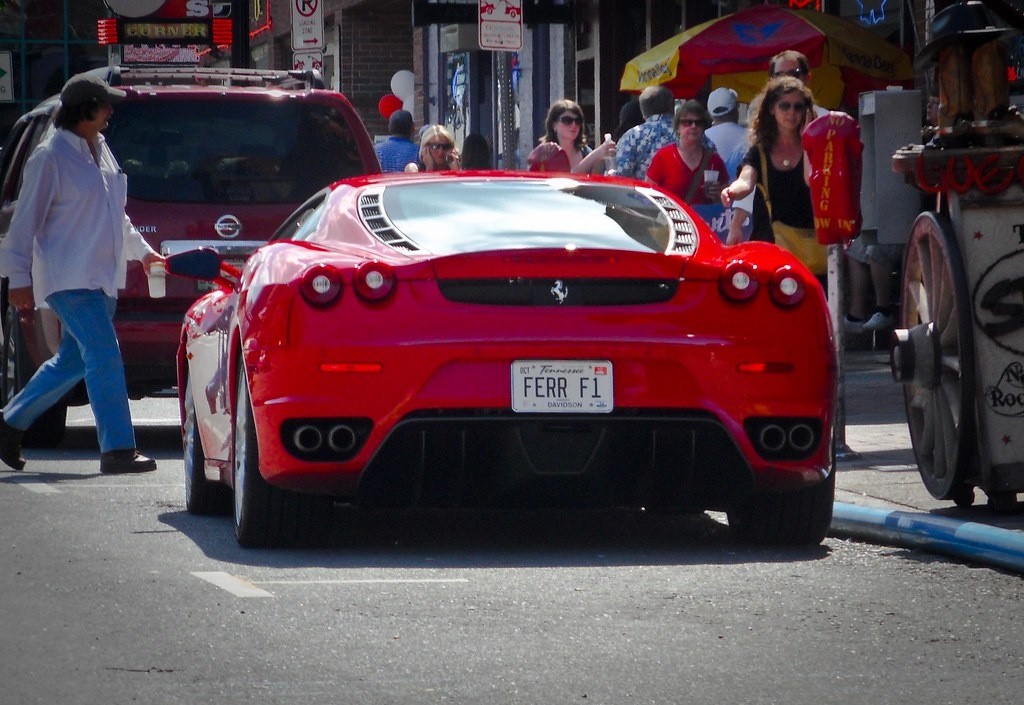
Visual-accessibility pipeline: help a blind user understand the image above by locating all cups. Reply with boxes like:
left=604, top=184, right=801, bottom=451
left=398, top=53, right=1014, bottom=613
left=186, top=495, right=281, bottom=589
left=147, top=261, right=166, bottom=298
left=703, top=170, right=719, bottom=198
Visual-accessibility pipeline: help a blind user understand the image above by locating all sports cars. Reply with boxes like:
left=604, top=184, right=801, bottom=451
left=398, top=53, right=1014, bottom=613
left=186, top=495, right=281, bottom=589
left=173, top=168, right=838, bottom=554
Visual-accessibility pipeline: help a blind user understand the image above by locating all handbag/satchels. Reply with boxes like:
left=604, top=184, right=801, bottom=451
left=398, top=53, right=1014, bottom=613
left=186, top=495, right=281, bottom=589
left=773, top=220, right=829, bottom=276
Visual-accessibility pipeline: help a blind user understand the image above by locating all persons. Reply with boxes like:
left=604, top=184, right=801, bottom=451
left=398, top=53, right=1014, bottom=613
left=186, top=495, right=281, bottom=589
left=526, top=50, right=941, bottom=330
left=0, top=74, right=167, bottom=473
left=374, top=111, right=492, bottom=176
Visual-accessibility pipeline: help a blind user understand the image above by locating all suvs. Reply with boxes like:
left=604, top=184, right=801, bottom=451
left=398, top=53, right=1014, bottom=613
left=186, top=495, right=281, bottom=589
left=0, top=65, right=382, bottom=449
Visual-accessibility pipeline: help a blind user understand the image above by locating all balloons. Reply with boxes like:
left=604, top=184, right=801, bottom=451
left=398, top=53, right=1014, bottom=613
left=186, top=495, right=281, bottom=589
left=379, top=70, right=415, bottom=121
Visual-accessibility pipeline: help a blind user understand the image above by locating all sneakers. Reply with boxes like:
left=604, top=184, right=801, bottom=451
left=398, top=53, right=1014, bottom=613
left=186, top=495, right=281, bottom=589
left=845, top=315, right=864, bottom=334
left=100, top=446, right=157, bottom=475
left=861, top=311, right=896, bottom=331
left=0, top=413, right=26, bottom=471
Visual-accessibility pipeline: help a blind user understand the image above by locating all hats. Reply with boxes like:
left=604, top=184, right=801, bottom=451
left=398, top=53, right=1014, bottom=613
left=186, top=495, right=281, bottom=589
left=389, top=110, right=411, bottom=129
left=61, top=75, right=126, bottom=107
left=707, top=89, right=738, bottom=118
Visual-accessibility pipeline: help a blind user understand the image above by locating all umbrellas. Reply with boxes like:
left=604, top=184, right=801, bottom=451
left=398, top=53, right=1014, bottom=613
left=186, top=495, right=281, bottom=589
left=618, top=4, right=917, bottom=114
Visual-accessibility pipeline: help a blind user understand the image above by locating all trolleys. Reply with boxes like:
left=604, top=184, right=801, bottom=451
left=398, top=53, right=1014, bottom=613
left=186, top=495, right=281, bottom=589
left=890, top=0, right=1024, bottom=508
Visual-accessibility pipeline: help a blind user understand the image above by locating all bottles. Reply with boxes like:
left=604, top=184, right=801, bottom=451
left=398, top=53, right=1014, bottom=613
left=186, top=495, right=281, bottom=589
left=603, top=133, right=618, bottom=172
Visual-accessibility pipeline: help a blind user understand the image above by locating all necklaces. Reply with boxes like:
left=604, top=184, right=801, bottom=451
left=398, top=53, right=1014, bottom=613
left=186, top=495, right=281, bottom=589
left=782, top=158, right=791, bottom=166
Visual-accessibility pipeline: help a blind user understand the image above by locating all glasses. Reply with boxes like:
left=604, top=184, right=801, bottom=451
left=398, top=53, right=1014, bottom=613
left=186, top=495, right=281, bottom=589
left=427, top=143, right=454, bottom=151
left=678, top=119, right=706, bottom=127
left=775, top=100, right=805, bottom=113
left=773, top=66, right=804, bottom=81
left=557, top=115, right=584, bottom=126
